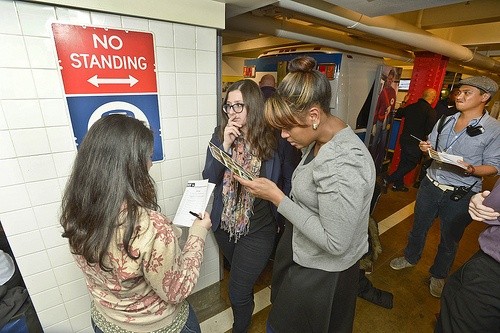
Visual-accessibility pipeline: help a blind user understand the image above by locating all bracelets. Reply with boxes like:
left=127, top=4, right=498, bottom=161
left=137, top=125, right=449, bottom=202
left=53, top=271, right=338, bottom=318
left=465, top=165, right=471, bottom=172
left=401, top=101, right=406, bottom=105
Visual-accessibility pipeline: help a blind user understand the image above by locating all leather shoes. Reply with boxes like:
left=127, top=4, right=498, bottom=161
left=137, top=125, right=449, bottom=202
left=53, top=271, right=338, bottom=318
left=392, top=185, right=409, bottom=192
left=381, top=174, right=389, bottom=194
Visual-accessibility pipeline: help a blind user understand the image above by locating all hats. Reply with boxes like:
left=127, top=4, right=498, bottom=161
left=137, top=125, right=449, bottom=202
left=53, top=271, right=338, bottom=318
left=459, top=75, right=498, bottom=95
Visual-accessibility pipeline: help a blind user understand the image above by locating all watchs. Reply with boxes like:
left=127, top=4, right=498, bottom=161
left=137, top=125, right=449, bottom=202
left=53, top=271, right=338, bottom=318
left=465, top=166, right=475, bottom=176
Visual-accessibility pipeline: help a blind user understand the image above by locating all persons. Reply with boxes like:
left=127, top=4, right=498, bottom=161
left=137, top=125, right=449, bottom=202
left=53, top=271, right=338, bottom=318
left=58, top=113, right=213, bottom=332
left=202, top=56, right=500, bottom=333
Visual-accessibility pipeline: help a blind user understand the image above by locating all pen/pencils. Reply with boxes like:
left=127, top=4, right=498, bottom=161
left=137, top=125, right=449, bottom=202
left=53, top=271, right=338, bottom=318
left=188, top=210, right=203, bottom=220
left=410, top=134, right=434, bottom=149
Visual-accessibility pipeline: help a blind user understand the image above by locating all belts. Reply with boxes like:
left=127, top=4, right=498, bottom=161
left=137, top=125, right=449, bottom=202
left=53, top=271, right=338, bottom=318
left=426, top=173, right=458, bottom=192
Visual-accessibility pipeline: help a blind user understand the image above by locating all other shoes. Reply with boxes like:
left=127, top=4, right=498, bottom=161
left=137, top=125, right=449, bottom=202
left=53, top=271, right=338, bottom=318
left=430, top=276, right=443, bottom=298
left=414, top=183, right=419, bottom=188
left=390, top=256, right=416, bottom=270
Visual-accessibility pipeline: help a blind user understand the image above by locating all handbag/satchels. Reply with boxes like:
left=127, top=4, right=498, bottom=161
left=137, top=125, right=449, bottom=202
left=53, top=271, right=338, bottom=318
left=421, top=158, right=433, bottom=178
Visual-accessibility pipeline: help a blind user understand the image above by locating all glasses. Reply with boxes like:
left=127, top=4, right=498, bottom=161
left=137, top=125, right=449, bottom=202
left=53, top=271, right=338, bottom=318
left=222, top=103, right=245, bottom=113
